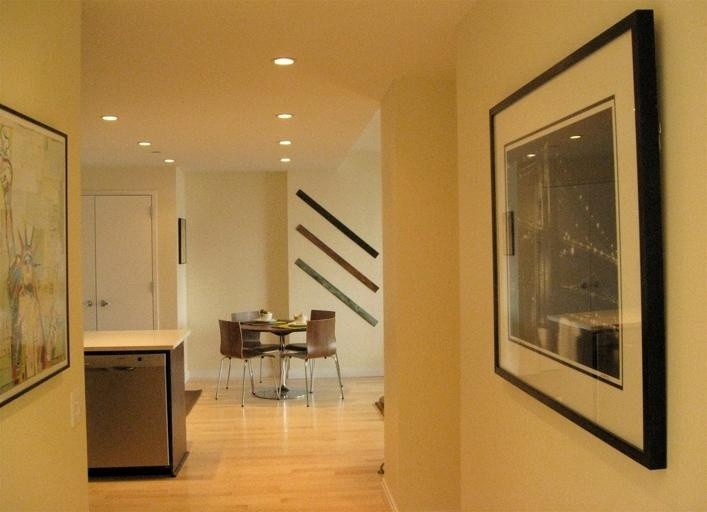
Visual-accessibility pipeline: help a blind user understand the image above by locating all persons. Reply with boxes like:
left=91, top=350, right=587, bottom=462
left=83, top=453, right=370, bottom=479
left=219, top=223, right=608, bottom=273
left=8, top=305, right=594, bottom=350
left=4, top=183, right=59, bottom=385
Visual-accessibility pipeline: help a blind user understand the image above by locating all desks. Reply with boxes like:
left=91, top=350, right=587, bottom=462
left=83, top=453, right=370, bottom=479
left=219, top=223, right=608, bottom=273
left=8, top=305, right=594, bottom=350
left=238, top=319, right=307, bottom=400
left=84, top=329, right=192, bottom=479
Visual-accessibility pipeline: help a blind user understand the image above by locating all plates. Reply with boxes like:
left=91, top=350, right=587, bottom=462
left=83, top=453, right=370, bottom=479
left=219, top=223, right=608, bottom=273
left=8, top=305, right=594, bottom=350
left=254, top=319, right=275, bottom=323
left=290, top=321, right=307, bottom=326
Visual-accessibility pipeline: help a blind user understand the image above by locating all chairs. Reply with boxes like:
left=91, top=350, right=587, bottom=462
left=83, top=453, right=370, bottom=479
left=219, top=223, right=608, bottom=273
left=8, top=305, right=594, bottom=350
left=226, top=311, right=280, bottom=401
left=282, top=310, right=343, bottom=393
left=278, top=317, right=345, bottom=407
left=215, top=319, right=281, bottom=407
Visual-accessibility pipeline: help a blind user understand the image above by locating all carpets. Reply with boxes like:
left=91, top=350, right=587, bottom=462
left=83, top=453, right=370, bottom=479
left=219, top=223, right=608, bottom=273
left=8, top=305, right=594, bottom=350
left=183, top=389, right=203, bottom=416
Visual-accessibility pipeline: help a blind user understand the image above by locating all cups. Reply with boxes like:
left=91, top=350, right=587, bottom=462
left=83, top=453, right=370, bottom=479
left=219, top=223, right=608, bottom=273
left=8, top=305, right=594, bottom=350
left=261, top=312, right=272, bottom=320
left=293, top=312, right=304, bottom=323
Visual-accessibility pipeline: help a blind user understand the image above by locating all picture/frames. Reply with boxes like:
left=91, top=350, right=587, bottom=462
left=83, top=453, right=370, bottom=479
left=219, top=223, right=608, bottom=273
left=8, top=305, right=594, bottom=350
left=178, top=218, right=188, bottom=264
left=488, top=9, right=667, bottom=470
left=0, top=103, right=70, bottom=408
